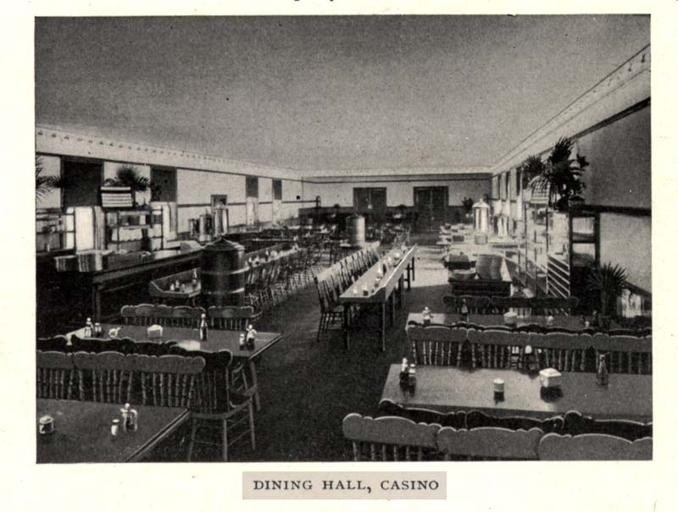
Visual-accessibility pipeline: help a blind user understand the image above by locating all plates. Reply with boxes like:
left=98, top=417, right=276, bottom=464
left=455, top=271, right=476, bottom=279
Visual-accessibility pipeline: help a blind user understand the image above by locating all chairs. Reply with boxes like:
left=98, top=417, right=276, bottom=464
left=36, top=229, right=418, bottom=462
left=342, top=296, right=653, bottom=459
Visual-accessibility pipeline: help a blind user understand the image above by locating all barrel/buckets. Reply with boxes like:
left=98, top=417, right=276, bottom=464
left=200, top=238, right=246, bottom=314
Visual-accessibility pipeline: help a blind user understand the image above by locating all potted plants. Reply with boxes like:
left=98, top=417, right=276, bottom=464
left=98, top=166, right=148, bottom=210
left=586, top=260, right=630, bottom=315
left=517, top=135, right=590, bottom=211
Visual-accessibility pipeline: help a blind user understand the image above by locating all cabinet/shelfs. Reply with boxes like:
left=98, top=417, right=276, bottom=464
left=518, top=201, right=600, bottom=308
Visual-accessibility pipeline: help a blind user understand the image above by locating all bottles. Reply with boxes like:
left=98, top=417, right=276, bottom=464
left=240, top=324, right=257, bottom=350
left=110, top=403, right=138, bottom=436
left=169, top=276, right=185, bottom=292
left=399, top=358, right=417, bottom=393
left=596, top=355, right=608, bottom=386
left=461, top=299, right=468, bottom=322
left=199, top=313, right=207, bottom=341
left=83, top=317, right=103, bottom=339
left=191, top=269, right=197, bottom=287
left=423, top=306, right=432, bottom=323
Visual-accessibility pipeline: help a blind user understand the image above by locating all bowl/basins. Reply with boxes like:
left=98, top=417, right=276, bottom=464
left=539, top=368, right=562, bottom=389
left=147, top=324, right=162, bottom=338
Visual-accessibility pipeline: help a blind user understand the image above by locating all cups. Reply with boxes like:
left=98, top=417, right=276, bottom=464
left=108, top=328, right=118, bottom=338
left=39, top=415, right=55, bottom=435
left=493, top=378, right=505, bottom=393
left=503, top=311, right=518, bottom=325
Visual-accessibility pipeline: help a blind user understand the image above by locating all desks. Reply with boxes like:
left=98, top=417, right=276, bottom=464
left=448, top=246, right=513, bottom=311
